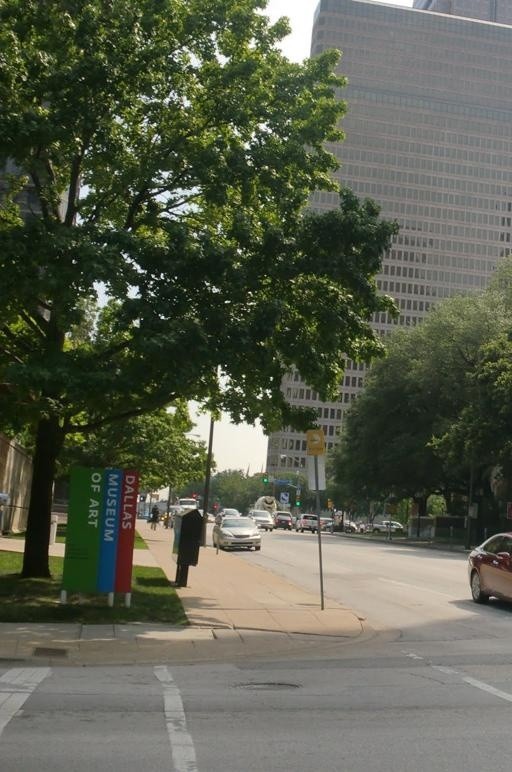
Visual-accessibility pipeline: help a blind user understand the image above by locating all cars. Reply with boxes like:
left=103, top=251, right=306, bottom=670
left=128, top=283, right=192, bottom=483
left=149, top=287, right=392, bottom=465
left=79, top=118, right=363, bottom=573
left=212, top=508, right=275, bottom=552
left=198, top=509, right=216, bottom=523
left=467, top=532, right=512, bottom=607
left=272, top=510, right=408, bottom=535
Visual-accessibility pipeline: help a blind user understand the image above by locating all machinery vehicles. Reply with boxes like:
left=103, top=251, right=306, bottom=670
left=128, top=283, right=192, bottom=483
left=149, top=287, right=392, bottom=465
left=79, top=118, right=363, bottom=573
left=255, top=495, right=277, bottom=515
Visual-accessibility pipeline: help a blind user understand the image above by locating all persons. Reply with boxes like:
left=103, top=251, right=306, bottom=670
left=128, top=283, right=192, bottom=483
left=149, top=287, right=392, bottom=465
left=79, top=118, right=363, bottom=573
left=151, top=504, right=159, bottom=531
left=213, top=511, right=234, bottom=555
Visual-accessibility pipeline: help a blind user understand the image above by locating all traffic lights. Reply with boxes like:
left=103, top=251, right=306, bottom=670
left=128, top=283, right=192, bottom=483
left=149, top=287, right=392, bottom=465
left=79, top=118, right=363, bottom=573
left=295, top=501, right=301, bottom=506
left=262, top=478, right=268, bottom=484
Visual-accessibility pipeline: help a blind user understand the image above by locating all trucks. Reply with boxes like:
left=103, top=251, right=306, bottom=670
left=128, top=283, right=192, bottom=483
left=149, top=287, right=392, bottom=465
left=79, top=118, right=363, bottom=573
left=177, top=498, right=197, bottom=516
